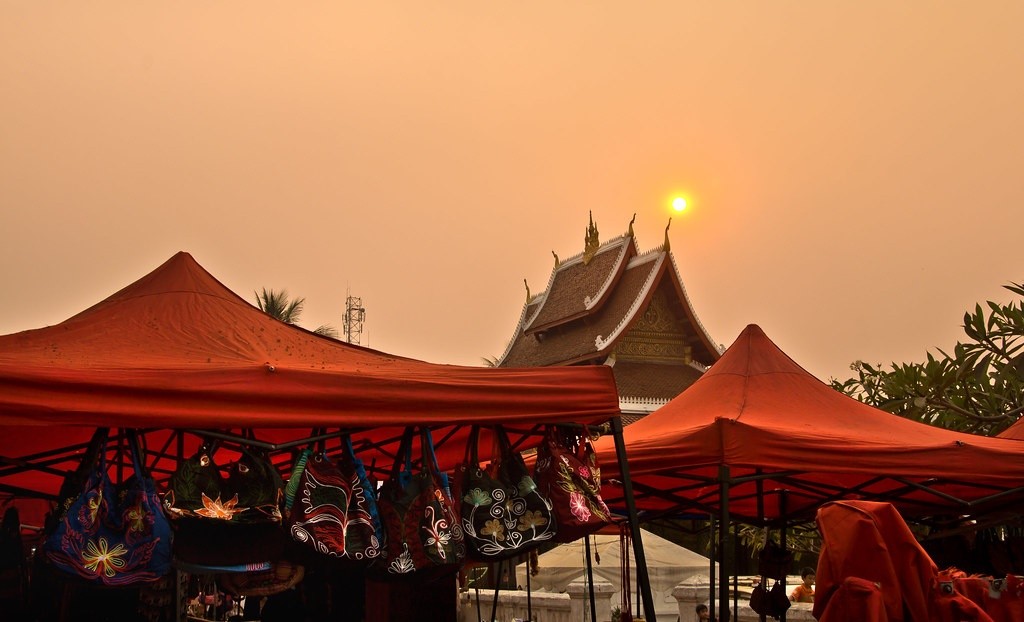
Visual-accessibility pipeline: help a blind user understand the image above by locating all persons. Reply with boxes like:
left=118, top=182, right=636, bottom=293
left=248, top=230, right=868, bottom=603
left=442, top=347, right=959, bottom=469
left=788, top=567, right=816, bottom=603
left=695, top=604, right=708, bottom=621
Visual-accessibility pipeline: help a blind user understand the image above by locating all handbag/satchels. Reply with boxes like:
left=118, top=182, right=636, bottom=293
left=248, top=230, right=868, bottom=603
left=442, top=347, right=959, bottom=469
left=749, top=580, right=792, bottom=617
left=41, top=421, right=613, bottom=621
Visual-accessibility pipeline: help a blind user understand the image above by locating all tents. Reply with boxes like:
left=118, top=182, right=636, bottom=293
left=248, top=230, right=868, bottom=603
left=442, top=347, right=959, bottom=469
left=468, top=324, right=1024, bottom=622
left=0, top=250, right=660, bottom=622
